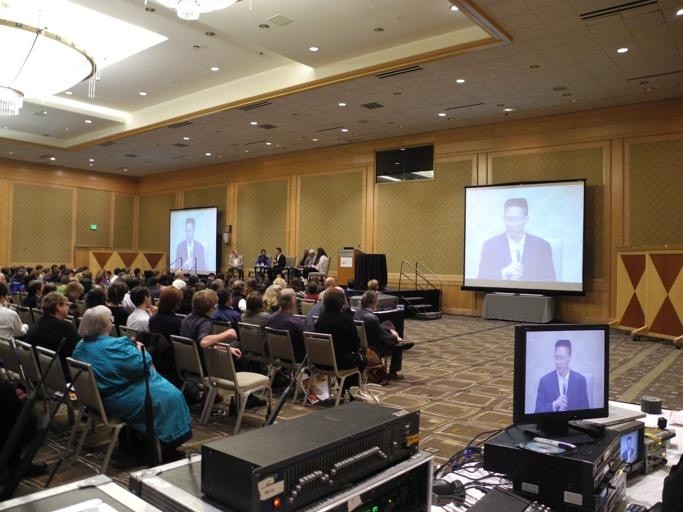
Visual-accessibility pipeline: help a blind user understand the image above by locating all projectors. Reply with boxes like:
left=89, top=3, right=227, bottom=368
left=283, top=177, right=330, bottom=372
left=350, top=294, right=399, bottom=312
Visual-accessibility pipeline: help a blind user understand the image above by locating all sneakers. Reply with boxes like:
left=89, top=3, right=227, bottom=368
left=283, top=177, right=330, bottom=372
left=245, top=393, right=267, bottom=410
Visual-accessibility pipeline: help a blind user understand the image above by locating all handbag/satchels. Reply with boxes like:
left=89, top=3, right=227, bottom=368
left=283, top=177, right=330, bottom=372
left=350, top=386, right=379, bottom=407
left=362, top=347, right=387, bottom=383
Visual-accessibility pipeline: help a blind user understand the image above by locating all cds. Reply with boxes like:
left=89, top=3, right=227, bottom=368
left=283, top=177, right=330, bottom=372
left=527, top=442, right=566, bottom=454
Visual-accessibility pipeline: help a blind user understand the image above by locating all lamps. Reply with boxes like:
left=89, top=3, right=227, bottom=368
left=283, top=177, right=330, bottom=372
left=0, top=14, right=96, bottom=120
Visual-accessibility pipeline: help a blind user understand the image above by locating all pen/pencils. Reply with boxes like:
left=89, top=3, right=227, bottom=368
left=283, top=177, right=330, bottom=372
left=533, top=437, right=575, bottom=451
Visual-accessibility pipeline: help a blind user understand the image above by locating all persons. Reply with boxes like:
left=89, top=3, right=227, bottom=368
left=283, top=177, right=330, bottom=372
left=478, top=198, right=556, bottom=281
left=622, top=436, right=634, bottom=462
left=175, top=217, right=205, bottom=271
left=535, top=340, right=589, bottom=413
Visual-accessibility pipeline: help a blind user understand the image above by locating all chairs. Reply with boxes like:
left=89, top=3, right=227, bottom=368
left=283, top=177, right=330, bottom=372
left=0, top=257, right=388, bottom=436
left=2, top=335, right=163, bottom=477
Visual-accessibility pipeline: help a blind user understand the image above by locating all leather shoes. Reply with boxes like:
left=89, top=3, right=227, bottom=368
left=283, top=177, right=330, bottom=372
left=395, top=342, right=415, bottom=350
left=388, top=373, right=406, bottom=381
left=18, top=460, right=48, bottom=476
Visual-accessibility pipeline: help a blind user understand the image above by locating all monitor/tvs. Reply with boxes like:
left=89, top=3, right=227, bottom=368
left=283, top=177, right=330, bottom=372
left=344, top=247, right=354, bottom=249
left=514, top=324, right=610, bottom=448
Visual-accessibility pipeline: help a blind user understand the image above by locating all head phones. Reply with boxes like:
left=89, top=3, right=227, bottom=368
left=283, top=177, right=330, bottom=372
left=432, top=479, right=465, bottom=507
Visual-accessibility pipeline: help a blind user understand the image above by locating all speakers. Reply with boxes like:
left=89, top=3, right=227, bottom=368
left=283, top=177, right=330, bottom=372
left=224, top=225, right=231, bottom=233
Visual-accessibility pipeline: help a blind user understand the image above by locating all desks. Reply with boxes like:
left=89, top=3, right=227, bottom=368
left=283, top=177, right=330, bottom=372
left=479, top=292, right=554, bottom=323
